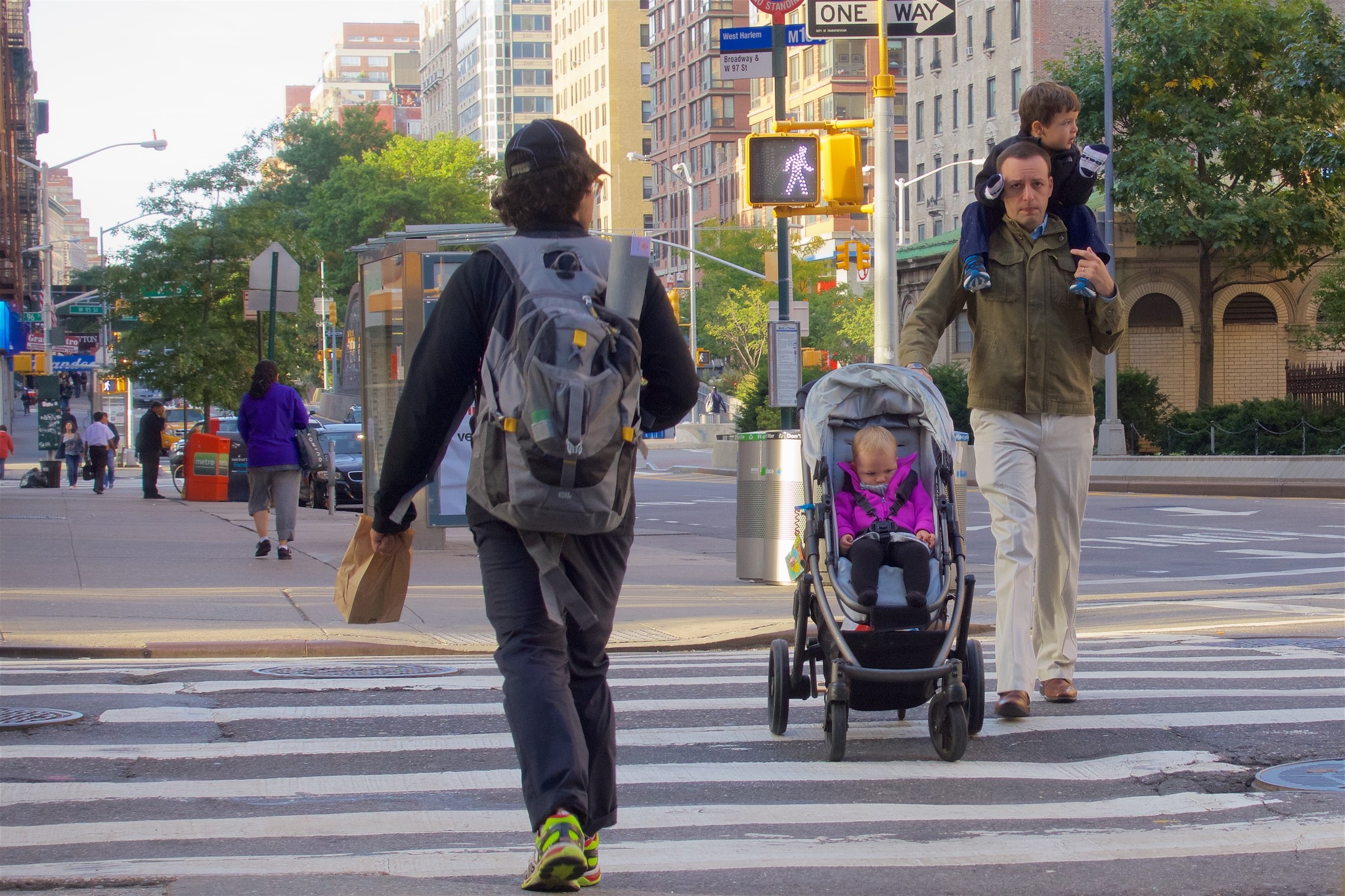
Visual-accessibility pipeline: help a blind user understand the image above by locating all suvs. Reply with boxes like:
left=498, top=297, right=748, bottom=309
left=298, top=423, right=363, bottom=509
left=159, top=405, right=208, bottom=455
left=170, top=415, right=242, bottom=478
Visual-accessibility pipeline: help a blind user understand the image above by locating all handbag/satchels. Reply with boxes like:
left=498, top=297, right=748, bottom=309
left=83, top=461, right=95, bottom=481
left=296, top=424, right=328, bottom=471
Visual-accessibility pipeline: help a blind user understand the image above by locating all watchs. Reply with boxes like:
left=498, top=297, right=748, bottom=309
left=907, top=363, right=928, bottom=372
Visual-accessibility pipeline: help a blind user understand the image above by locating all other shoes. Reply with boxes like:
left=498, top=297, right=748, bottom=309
left=93, top=488, right=104, bottom=494
left=144, top=494, right=165, bottom=499
left=858, top=590, right=878, bottom=606
left=905, top=591, right=927, bottom=607
left=104, top=481, right=113, bottom=489
left=69, top=483, right=76, bottom=489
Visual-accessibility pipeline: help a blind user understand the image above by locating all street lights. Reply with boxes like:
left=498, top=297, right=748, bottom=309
left=861, top=157, right=988, bottom=245
left=626, top=151, right=699, bottom=423
left=0, top=137, right=186, bottom=467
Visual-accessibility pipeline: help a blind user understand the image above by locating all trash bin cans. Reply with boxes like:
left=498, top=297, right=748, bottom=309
left=762, top=429, right=826, bottom=588
left=38, top=459, right=62, bottom=488
left=733, top=431, right=768, bottom=584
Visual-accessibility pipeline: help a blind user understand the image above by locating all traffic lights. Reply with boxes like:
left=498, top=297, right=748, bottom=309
left=745, top=132, right=820, bottom=209
left=835, top=243, right=850, bottom=271
left=697, top=349, right=710, bottom=364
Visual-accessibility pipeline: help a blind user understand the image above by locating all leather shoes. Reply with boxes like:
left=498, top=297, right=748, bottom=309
left=995, top=690, right=1030, bottom=717
left=1039, top=678, right=1078, bottom=703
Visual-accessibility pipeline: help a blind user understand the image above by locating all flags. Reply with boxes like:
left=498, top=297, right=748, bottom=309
left=677, top=274, right=684, bottom=283
left=667, top=276, right=674, bottom=288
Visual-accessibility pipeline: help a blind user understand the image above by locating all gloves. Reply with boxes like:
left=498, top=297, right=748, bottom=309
left=1080, top=143, right=1110, bottom=178
left=984, top=174, right=1004, bottom=200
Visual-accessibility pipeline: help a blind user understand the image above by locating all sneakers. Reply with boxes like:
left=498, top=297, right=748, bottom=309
left=521, top=814, right=588, bottom=891
left=1070, top=278, right=1096, bottom=298
left=963, top=255, right=991, bottom=293
left=577, top=831, right=601, bottom=886
left=278, top=545, right=292, bottom=559
left=255, top=539, right=271, bottom=556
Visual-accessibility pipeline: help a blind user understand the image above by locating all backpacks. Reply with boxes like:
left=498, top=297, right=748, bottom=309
left=463, top=236, right=643, bottom=535
left=705, top=393, right=714, bottom=412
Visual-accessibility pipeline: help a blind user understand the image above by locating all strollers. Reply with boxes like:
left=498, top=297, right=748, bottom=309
left=765, top=361, right=983, bottom=761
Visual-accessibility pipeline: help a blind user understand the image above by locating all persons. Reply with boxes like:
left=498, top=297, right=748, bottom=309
left=139, top=400, right=165, bottom=499
left=81, top=412, right=116, bottom=494
left=365, top=118, right=697, bottom=893
left=53, top=369, right=87, bottom=413
left=21, top=390, right=31, bottom=416
left=61, top=407, right=78, bottom=433
left=704, top=386, right=727, bottom=416
left=54, top=420, right=85, bottom=490
left=835, top=423, right=936, bottom=607
left=897, top=141, right=1125, bottom=719
left=135, top=432, right=139, bottom=462
left=957, top=80, right=1111, bottom=299
left=0, top=425, right=14, bottom=481
left=237, top=358, right=309, bottom=559
left=101, top=412, right=120, bottom=490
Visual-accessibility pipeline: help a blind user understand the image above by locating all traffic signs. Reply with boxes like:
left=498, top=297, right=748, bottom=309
left=805, top=0, right=960, bottom=40
left=720, top=22, right=826, bottom=80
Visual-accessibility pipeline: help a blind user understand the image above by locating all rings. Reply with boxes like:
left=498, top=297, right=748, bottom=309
left=1082, top=267, right=1085, bottom=272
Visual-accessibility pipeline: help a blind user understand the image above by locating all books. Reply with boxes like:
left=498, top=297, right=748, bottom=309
left=785, top=543, right=811, bottom=582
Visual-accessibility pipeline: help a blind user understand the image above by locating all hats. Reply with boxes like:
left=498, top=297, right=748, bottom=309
left=712, top=386, right=717, bottom=389
left=505, top=119, right=613, bottom=182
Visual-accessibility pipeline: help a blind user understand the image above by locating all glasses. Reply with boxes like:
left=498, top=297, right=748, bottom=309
left=591, top=178, right=604, bottom=199
